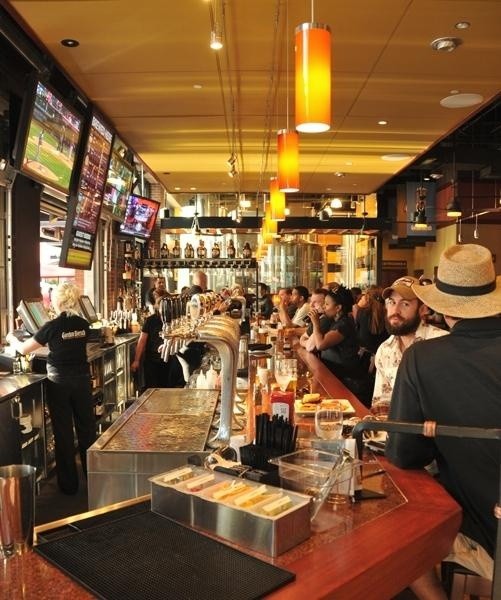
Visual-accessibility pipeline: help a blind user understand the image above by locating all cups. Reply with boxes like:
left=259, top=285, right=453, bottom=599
left=303, top=306, right=325, bottom=324
left=274, top=359, right=292, bottom=392
left=315, top=401, right=344, bottom=441
left=12, top=360, right=32, bottom=375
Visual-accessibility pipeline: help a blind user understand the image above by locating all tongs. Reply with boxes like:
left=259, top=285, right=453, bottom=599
left=310, top=448, right=355, bottom=522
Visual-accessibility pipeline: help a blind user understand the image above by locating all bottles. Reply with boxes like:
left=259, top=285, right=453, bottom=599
left=91, top=376, right=96, bottom=391
left=197, top=364, right=218, bottom=389
left=283, top=337, right=291, bottom=353
left=1, top=464, right=36, bottom=558
left=110, top=311, right=130, bottom=334
left=147, top=240, right=252, bottom=258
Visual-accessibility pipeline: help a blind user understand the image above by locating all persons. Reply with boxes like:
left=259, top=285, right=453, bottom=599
left=34, top=126, right=75, bottom=161
left=132, top=271, right=227, bottom=396
left=6, top=282, right=96, bottom=494
left=220, top=282, right=389, bottom=407
left=372, top=244, right=500, bottom=598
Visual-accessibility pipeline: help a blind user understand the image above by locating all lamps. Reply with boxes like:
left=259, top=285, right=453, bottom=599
left=474, top=214, right=480, bottom=241
left=457, top=218, right=464, bottom=244
left=190, top=0, right=367, bottom=263
left=415, top=175, right=428, bottom=228
left=446, top=155, right=462, bottom=216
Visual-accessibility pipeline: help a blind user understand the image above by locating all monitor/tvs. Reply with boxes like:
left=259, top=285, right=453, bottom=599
left=101, top=149, right=133, bottom=221
left=115, top=194, right=160, bottom=242
left=15, top=79, right=87, bottom=197
left=78, top=294, right=98, bottom=323
left=15, top=297, right=52, bottom=335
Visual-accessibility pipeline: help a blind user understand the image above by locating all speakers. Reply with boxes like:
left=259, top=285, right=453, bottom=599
left=351, top=195, right=360, bottom=203
left=160, top=208, right=170, bottom=220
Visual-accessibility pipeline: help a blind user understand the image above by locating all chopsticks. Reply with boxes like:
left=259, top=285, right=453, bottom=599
left=239, top=412, right=299, bottom=471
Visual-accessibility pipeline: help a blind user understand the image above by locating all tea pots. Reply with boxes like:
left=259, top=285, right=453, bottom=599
left=130, top=322, right=140, bottom=333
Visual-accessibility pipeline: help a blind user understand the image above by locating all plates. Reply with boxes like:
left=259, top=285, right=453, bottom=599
left=19, top=412, right=33, bottom=434
left=294, top=399, right=356, bottom=417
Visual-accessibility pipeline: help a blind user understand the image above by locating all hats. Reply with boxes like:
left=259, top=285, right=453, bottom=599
left=410, top=243, right=501, bottom=319
left=381, top=275, right=423, bottom=301
